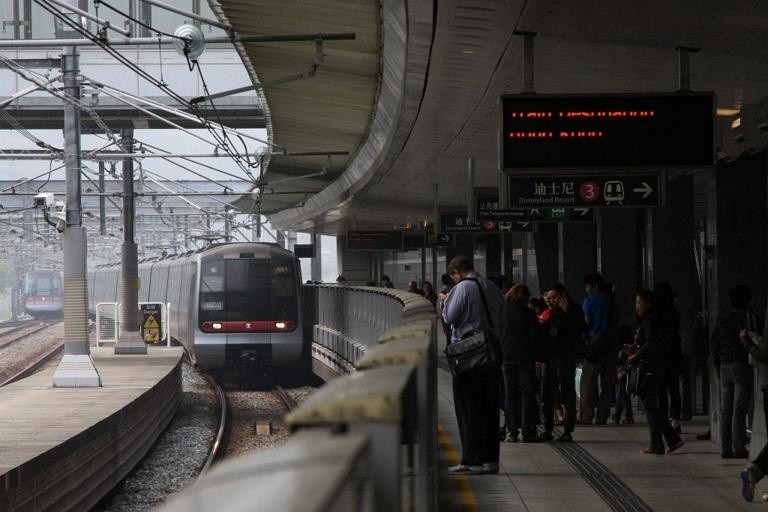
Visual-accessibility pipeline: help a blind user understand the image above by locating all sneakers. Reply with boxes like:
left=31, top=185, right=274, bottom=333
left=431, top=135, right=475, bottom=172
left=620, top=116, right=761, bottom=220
left=667, top=441, right=684, bottom=453
left=448, top=462, right=499, bottom=474
left=507, top=431, right=572, bottom=443
left=640, top=448, right=665, bottom=456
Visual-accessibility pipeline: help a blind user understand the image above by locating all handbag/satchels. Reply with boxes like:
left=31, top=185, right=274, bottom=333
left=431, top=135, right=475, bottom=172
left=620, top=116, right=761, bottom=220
left=746, top=312, right=764, bottom=370
left=446, top=331, right=503, bottom=381
left=625, top=367, right=644, bottom=395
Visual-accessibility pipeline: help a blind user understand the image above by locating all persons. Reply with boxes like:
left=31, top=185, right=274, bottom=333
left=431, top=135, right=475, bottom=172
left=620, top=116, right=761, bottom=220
left=440, top=271, right=461, bottom=337
left=737, top=294, right=768, bottom=503
left=380, top=276, right=392, bottom=288
left=407, top=281, right=420, bottom=293
left=420, top=281, right=438, bottom=310
left=711, top=284, right=764, bottom=457
left=488, top=274, right=709, bottom=457
left=337, top=276, right=350, bottom=285
left=438, top=255, right=510, bottom=476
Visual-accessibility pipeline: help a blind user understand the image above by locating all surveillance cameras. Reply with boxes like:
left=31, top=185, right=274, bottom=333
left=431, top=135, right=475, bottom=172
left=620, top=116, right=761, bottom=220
left=33, top=192, right=54, bottom=207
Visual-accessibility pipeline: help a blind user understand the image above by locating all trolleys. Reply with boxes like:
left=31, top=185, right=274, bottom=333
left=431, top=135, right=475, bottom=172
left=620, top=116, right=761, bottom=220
left=498, top=376, right=544, bottom=443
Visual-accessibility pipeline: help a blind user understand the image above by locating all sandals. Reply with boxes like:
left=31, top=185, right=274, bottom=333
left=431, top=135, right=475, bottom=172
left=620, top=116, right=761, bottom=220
left=741, top=468, right=755, bottom=502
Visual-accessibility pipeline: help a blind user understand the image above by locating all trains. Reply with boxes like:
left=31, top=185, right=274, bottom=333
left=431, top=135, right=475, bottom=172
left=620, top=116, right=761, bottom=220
left=18, top=268, right=65, bottom=319
left=83, top=238, right=303, bottom=382
